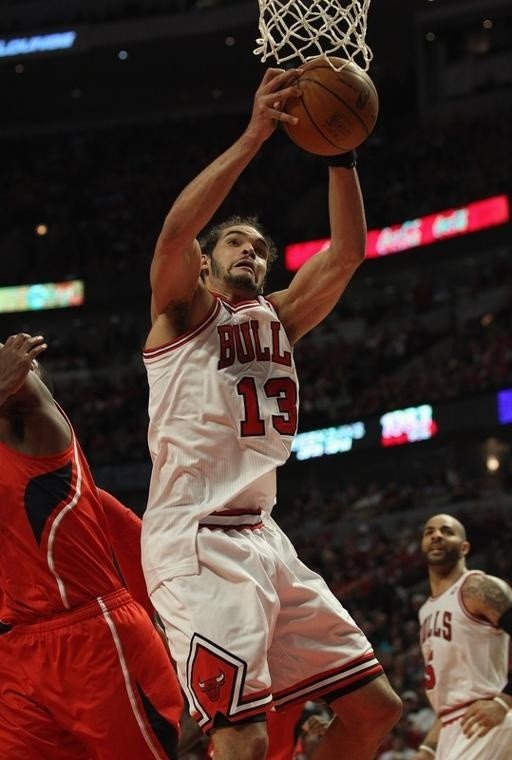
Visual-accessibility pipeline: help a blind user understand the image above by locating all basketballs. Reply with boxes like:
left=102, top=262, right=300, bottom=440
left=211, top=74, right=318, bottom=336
left=282, top=56, right=380, bottom=157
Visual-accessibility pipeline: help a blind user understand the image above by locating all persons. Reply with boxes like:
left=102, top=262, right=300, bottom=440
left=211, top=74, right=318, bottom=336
left=133, top=56, right=409, bottom=757
left=2, top=332, right=196, bottom=758
left=94, top=488, right=212, bottom=758
left=6, top=304, right=149, bottom=519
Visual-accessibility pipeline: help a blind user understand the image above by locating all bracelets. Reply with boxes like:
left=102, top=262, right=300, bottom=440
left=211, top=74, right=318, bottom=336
left=321, top=148, right=359, bottom=168
